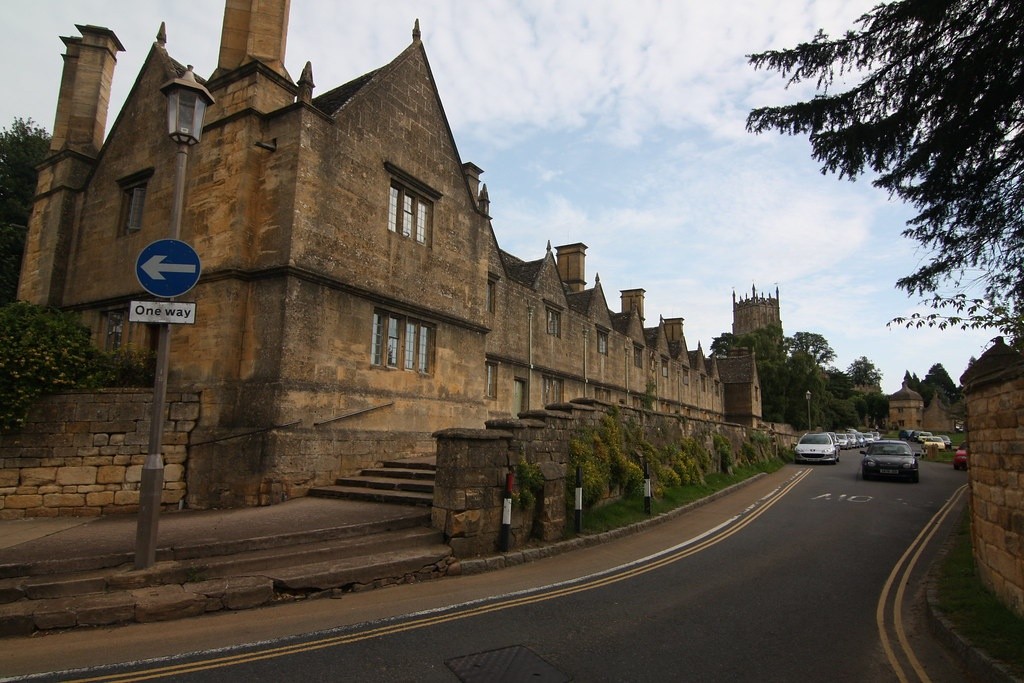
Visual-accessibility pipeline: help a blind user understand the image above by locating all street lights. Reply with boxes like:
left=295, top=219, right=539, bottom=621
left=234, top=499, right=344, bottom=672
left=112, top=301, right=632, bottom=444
left=805, top=390, right=811, bottom=432
left=130, top=62, right=214, bottom=572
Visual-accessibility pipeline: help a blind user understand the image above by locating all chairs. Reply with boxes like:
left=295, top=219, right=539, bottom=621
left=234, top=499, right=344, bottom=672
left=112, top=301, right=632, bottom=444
left=897, top=446, right=905, bottom=453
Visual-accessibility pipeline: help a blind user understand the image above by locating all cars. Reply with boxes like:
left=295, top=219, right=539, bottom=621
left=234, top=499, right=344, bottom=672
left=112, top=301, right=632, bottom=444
left=953, top=440, right=968, bottom=471
left=859, top=439, right=921, bottom=483
left=898, top=429, right=951, bottom=452
left=793, top=432, right=840, bottom=465
left=819, top=428, right=881, bottom=450
